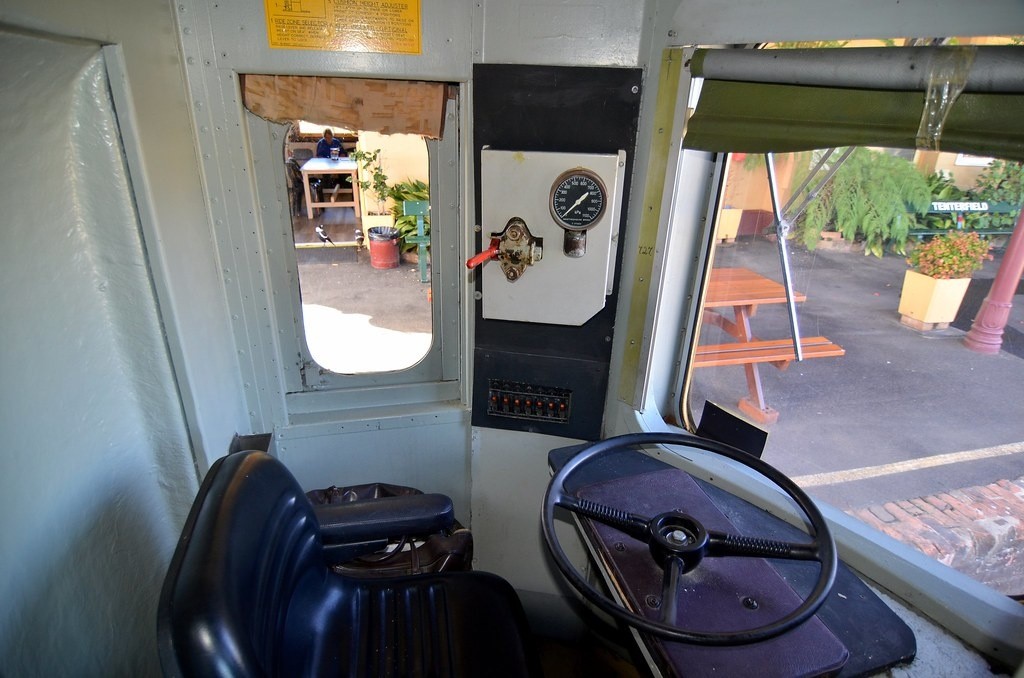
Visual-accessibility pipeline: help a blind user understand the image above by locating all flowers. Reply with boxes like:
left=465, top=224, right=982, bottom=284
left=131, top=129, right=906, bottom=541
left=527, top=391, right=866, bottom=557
left=904, top=229, right=994, bottom=280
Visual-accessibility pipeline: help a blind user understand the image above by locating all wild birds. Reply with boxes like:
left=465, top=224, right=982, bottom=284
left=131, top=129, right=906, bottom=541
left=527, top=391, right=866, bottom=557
left=315, top=227, right=336, bottom=247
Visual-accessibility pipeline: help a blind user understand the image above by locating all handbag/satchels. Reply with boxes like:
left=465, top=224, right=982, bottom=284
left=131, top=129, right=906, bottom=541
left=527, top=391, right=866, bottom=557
left=305, top=483, right=473, bottom=577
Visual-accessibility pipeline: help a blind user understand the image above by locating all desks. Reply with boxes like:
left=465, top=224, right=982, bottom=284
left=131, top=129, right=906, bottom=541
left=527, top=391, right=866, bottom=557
left=702, top=268, right=806, bottom=424
left=299, top=157, right=360, bottom=219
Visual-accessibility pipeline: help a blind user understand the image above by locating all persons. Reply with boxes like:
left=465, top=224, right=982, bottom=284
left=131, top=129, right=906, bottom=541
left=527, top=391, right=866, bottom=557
left=315, top=128, right=347, bottom=190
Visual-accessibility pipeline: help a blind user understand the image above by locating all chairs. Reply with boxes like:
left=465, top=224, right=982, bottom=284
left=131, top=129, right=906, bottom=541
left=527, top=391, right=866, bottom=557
left=157, top=449, right=536, bottom=678
left=285, top=157, right=326, bottom=217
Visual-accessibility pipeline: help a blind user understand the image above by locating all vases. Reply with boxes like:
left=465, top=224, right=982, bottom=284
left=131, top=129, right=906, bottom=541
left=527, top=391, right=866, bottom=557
left=897, top=268, right=973, bottom=331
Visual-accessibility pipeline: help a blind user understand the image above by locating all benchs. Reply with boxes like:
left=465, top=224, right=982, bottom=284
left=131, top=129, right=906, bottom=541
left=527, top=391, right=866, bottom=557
left=886, top=198, right=1022, bottom=260
left=402, top=201, right=430, bottom=282
left=693, top=336, right=846, bottom=366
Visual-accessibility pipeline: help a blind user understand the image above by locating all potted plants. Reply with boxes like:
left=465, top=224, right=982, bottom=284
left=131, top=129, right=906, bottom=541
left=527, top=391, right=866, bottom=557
left=345, top=148, right=404, bottom=269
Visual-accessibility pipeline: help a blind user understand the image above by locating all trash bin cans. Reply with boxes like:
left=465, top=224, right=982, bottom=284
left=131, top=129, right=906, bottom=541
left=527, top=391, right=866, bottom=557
left=368, top=226, right=400, bottom=269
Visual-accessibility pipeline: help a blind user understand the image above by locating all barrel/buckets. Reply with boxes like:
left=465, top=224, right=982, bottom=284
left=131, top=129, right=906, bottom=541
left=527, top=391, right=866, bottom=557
left=330, top=148, right=340, bottom=161
